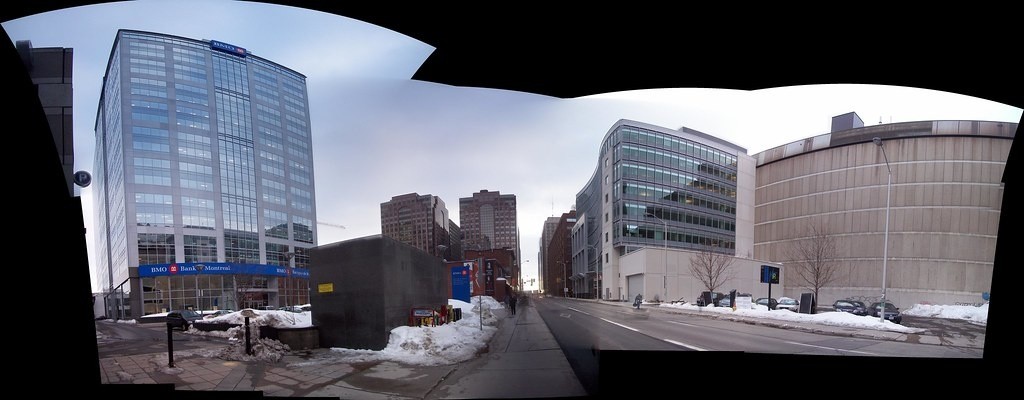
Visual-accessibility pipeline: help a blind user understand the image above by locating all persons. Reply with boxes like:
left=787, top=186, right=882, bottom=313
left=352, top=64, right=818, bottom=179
left=509, top=293, right=517, bottom=315
left=634, top=294, right=642, bottom=309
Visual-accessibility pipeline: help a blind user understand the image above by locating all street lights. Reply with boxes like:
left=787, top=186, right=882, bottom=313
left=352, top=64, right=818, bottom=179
left=871, top=136, right=892, bottom=323
left=195, top=264, right=205, bottom=308
left=586, top=244, right=599, bottom=303
left=644, top=212, right=668, bottom=304
left=557, top=261, right=567, bottom=298
left=284, top=251, right=296, bottom=311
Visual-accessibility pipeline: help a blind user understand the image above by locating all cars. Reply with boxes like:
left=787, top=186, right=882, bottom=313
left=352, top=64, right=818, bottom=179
left=774, top=298, right=800, bottom=314
left=719, top=295, right=731, bottom=307
left=697, top=291, right=723, bottom=307
left=167, top=309, right=203, bottom=331
left=755, top=297, right=778, bottom=310
left=867, top=300, right=902, bottom=324
left=833, top=300, right=869, bottom=317
